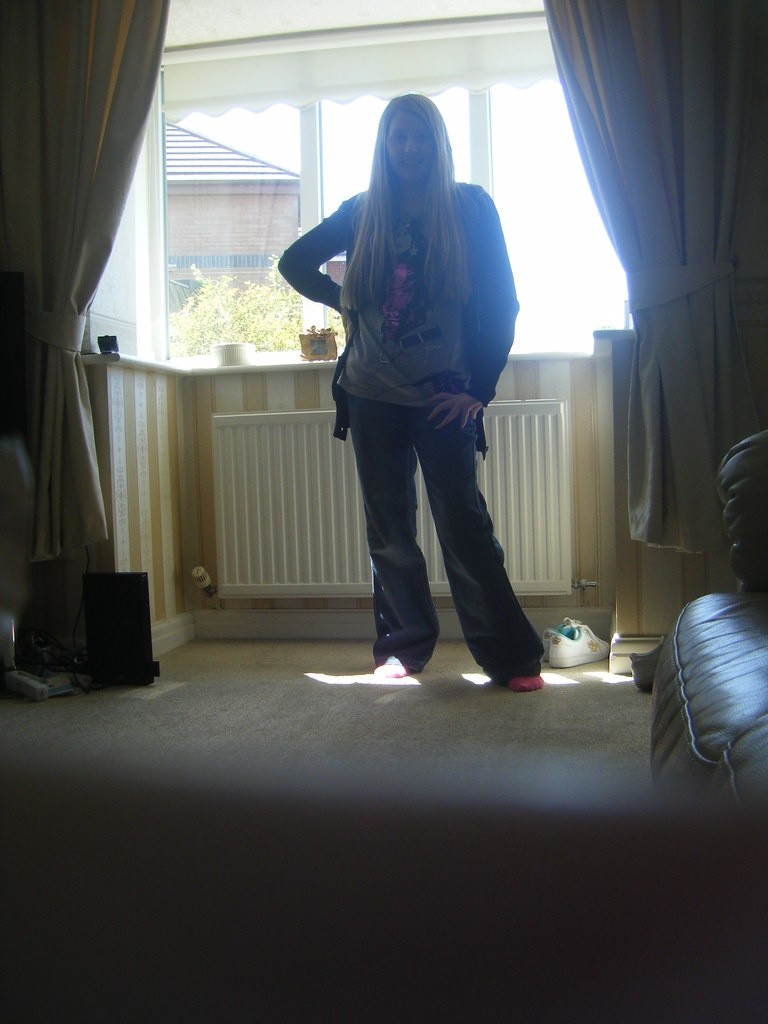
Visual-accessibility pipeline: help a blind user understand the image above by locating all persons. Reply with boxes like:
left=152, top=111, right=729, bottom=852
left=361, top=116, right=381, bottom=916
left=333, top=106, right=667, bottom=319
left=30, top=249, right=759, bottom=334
left=277, top=93, right=544, bottom=691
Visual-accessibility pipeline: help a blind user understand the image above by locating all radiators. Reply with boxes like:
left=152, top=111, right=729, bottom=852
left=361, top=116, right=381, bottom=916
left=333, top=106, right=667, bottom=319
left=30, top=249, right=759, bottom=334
left=210, top=398, right=574, bottom=597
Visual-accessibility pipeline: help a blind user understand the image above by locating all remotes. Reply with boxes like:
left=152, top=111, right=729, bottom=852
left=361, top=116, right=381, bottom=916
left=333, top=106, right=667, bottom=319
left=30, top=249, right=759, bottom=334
left=7, top=671, right=49, bottom=703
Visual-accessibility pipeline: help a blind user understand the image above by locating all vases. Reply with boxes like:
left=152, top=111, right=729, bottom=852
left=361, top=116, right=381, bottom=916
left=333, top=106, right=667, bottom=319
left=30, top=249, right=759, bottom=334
left=210, top=342, right=253, bottom=367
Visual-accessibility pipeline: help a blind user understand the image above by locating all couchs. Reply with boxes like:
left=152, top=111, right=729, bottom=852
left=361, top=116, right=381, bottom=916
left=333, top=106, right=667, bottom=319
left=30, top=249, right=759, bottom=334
left=652, top=426, right=768, bottom=812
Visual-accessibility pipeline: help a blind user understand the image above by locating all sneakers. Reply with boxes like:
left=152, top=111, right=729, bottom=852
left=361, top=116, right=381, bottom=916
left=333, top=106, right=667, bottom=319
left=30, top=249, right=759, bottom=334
left=549, top=623, right=610, bottom=668
left=543, top=618, right=582, bottom=661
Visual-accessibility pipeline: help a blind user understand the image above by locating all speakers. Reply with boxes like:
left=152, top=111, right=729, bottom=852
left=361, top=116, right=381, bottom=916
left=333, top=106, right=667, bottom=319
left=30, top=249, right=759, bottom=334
left=83, top=572, right=154, bottom=687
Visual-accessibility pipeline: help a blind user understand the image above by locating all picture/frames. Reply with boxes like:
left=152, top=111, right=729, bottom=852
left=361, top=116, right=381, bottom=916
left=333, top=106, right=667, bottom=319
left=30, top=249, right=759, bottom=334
left=298, top=326, right=339, bottom=361
